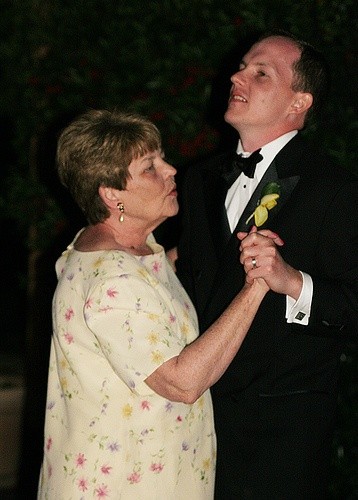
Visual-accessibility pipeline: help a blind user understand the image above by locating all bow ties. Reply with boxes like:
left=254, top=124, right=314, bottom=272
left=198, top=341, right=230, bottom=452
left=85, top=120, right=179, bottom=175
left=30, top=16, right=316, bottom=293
left=220, top=149, right=264, bottom=191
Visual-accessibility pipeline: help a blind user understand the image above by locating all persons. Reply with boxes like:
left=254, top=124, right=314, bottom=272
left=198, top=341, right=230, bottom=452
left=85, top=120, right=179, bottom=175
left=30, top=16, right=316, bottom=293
left=36, top=108, right=283, bottom=500
left=175, top=24, right=358, bottom=500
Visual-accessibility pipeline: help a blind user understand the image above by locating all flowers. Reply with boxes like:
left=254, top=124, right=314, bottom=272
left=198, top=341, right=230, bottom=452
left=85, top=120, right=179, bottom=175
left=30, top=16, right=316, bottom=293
left=246, top=182, right=280, bottom=227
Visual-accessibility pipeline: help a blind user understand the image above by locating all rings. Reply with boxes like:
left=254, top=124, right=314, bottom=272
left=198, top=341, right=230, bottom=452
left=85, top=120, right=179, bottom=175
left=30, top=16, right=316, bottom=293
left=251, top=255, right=257, bottom=269
